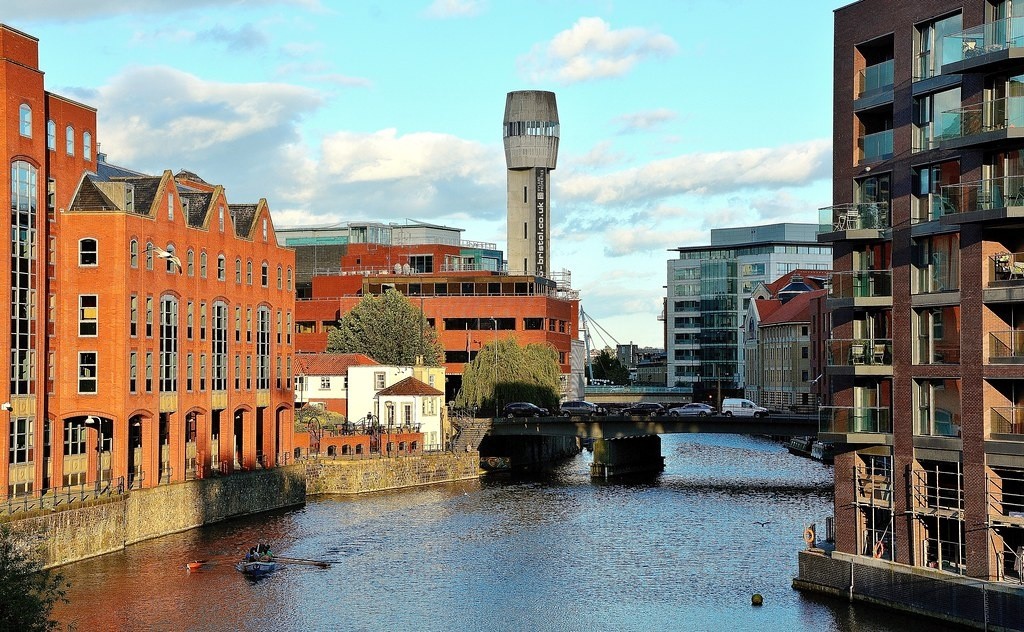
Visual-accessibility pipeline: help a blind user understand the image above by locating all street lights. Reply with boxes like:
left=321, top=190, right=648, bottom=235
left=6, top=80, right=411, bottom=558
left=724, top=372, right=729, bottom=398
left=488, top=318, right=499, bottom=418
left=696, top=372, right=701, bottom=402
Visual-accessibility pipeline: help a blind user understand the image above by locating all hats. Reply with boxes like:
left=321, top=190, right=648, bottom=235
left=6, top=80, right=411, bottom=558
left=246, top=553, right=251, bottom=558
left=254, top=552, right=259, bottom=557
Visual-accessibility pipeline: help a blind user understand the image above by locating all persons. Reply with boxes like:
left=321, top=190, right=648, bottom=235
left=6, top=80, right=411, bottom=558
left=873, top=541, right=884, bottom=558
left=244, top=538, right=273, bottom=562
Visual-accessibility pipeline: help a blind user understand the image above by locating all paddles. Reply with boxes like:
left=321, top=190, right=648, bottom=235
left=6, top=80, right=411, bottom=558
left=269, top=556, right=331, bottom=567
left=186, top=555, right=246, bottom=573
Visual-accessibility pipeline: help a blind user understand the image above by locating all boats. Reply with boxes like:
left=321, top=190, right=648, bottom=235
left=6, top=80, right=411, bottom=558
left=243, top=560, right=276, bottom=575
left=590, top=434, right=664, bottom=485
left=811, top=441, right=847, bottom=464
left=788, top=435, right=818, bottom=458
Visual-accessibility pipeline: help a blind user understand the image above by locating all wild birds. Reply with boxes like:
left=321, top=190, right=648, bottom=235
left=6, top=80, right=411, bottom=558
left=752, top=521, right=771, bottom=527
left=142, top=247, right=183, bottom=276
left=462, top=489, right=471, bottom=498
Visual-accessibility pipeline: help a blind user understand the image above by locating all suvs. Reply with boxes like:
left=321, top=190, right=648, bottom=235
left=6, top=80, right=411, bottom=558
left=502, top=402, right=550, bottom=419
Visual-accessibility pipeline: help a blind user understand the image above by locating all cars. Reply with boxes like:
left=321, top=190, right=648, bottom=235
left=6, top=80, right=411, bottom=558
left=668, top=403, right=718, bottom=418
left=619, top=403, right=665, bottom=417
left=560, top=401, right=608, bottom=418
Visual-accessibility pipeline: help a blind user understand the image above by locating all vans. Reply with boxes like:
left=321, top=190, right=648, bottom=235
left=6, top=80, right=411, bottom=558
left=722, top=398, right=769, bottom=418
